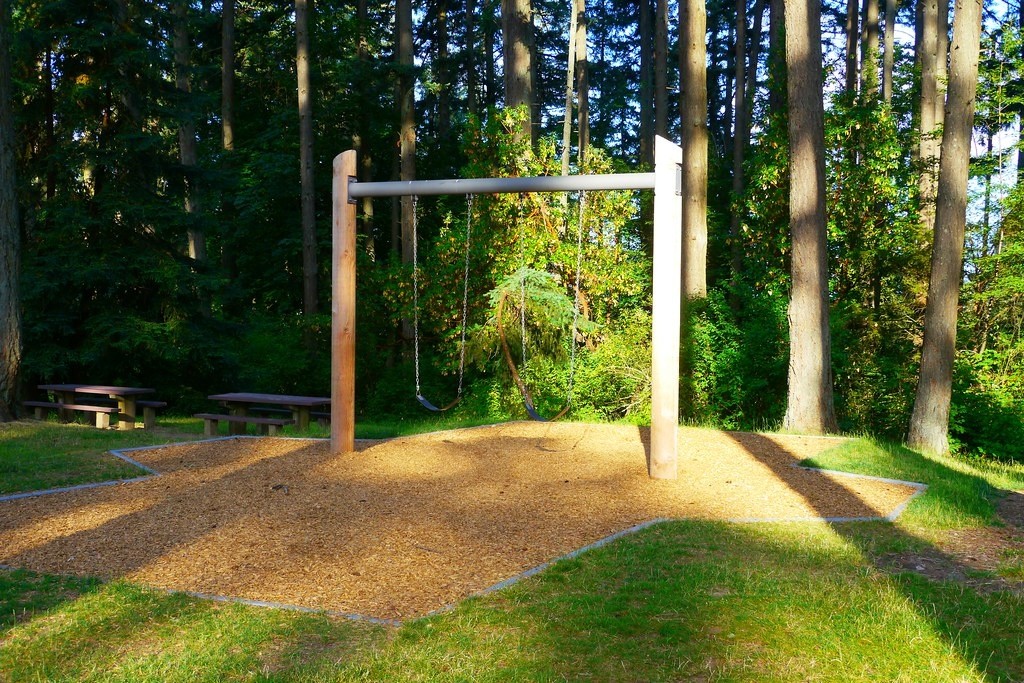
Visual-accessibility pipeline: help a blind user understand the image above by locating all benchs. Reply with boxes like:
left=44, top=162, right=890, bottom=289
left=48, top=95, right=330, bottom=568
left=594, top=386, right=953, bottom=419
left=73, top=397, right=167, bottom=429
left=193, top=413, right=296, bottom=437
left=21, top=400, right=121, bottom=429
left=247, top=406, right=331, bottom=431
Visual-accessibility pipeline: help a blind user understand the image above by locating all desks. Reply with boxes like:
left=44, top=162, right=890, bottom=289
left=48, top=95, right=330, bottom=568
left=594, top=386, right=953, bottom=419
left=37, top=384, right=155, bottom=431
left=208, top=393, right=332, bottom=431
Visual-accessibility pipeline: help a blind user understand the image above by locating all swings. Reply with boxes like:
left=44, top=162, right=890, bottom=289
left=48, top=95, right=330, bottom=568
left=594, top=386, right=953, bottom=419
left=519, top=196, right=585, bottom=422
left=412, top=195, right=472, bottom=413
